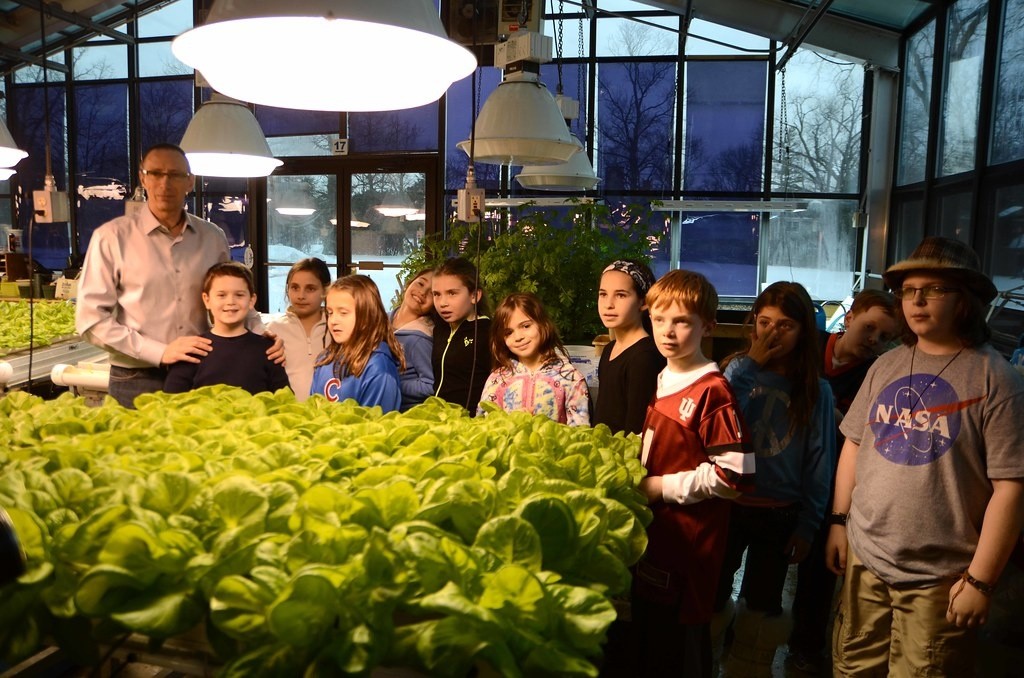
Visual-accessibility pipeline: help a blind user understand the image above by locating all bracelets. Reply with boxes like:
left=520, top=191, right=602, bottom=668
left=830, top=512, right=847, bottom=525
left=949, top=569, right=995, bottom=613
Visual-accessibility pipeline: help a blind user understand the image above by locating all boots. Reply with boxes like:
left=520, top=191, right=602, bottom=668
left=709, top=595, right=735, bottom=678
left=726, top=596, right=794, bottom=678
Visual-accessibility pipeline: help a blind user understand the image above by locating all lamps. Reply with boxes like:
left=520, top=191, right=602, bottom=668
left=276, top=182, right=316, bottom=215
left=178, top=92, right=284, bottom=177
left=0, top=115, right=29, bottom=168
left=373, top=189, right=419, bottom=217
left=651, top=60, right=809, bottom=212
left=451, top=8, right=606, bottom=208
left=172, top=0, right=478, bottom=112
left=456, top=0, right=581, bottom=167
left=515, top=0, right=602, bottom=192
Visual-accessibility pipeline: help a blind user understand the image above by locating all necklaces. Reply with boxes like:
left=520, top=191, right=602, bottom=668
left=905, top=341, right=965, bottom=426
left=169, top=217, right=183, bottom=229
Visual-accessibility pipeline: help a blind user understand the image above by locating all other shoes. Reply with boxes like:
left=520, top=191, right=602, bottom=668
left=785, top=638, right=818, bottom=673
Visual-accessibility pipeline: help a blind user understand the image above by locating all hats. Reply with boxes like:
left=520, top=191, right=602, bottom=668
left=884, top=237, right=998, bottom=305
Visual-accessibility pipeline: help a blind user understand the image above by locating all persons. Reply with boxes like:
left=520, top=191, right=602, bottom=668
left=591, top=258, right=667, bottom=438
left=775, top=288, right=903, bottom=614
left=73, top=143, right=286, bottom=410
left=386, top=268, right=435, bottom=414
left=266, top=256, right=331, bottom=401
left=431, top=257, right=491, bottom=417
left=718, top=281, right=835, bottom=678
left=475, top=292, right=591, bottom=428
left=310, top=273, right=406, bottom=415
left=638, top=270, right=755, bottom=678
left=825, top=235, right=1024, bottom=678
left=162, top=261, right=295, bottom=396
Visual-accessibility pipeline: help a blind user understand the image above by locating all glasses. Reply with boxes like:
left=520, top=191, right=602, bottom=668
left=139, top=164, right=190, bottom=181
left=893, top=284, right=973, bottom=300
